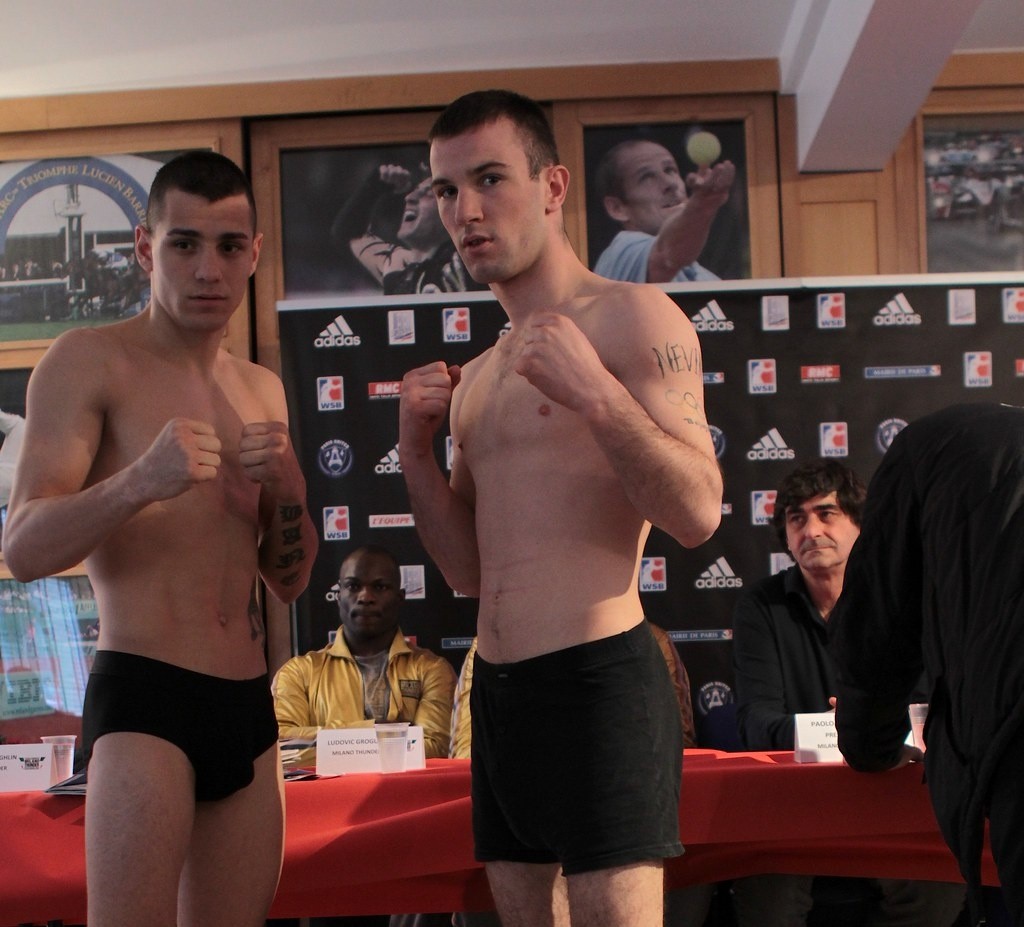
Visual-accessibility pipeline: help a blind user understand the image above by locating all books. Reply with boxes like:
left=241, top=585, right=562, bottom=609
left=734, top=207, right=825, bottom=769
left=280, top=739, right=316, bottom=769
left=44, top=769, right=88, bottom=796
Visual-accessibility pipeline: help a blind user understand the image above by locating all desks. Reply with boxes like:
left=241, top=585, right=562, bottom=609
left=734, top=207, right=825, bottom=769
left=0, top=750, right=1000, bottom=927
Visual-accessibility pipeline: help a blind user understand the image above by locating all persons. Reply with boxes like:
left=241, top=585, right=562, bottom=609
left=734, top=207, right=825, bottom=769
left=825, top=400, right=1023, bottom=927
left=271, top=544, right=457, bottom=926
left=4, top=147, right=319, bottom=927
left=733, top=457, right=968, bottom=927
left=0, top=249, right=139, bottom=320
left=397, top=88, right=721, bottom=927
left=447, top=619, right=716, bottom=927
left=330, top=164, right=491, bottom=296
left=592, top=138, right=737, bottom=285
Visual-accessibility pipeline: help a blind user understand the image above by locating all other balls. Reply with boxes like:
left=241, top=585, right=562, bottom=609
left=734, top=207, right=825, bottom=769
left=686, top=131, right=721, bottom=164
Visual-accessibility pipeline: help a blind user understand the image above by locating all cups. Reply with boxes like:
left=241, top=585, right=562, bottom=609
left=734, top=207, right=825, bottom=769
left=374, top=721, right=411, bottom=772
left=907, top=702, right=931, bottom=760
left=39, top=736, right=78, bottom=783
